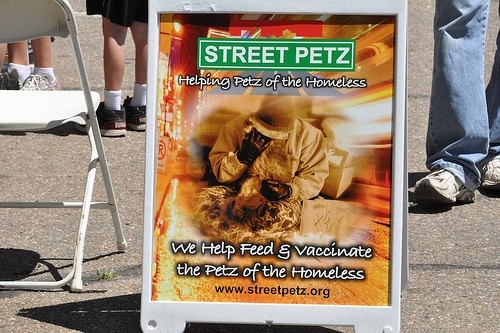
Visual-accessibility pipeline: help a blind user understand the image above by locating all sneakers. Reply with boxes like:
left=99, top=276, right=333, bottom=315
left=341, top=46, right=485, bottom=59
left=0, top=67, right=22, bottom=90
left=75, top=102, right=126, bottom=136
left=477, top=158, right=500, bottom=187
left=413, top=169, right=476, bottom=204
left=19, top=73, right=61, bottom=91
left=123, top=95, right=146, bottom=130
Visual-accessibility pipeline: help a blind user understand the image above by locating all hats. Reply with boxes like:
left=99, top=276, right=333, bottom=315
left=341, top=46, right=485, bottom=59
left=250, top=96, right=294, bottom=139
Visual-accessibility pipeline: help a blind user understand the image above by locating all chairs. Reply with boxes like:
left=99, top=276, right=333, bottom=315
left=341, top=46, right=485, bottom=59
left=0, top=0, right=127, bottom=293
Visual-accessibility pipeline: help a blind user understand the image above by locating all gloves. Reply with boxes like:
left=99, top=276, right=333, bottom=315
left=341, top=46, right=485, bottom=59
left=259, top=179, right=291, bottom=201
left=237, top=130, right=272, bottom=164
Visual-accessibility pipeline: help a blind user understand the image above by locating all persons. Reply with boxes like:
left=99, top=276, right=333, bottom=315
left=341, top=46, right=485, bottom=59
left=76, top=0, right=149, bottom=137
left=0, top=35, right=60, bottom=92
left=413, top=0, right=500, bottom=205
left=207, top=95, right=329, bottom=203
